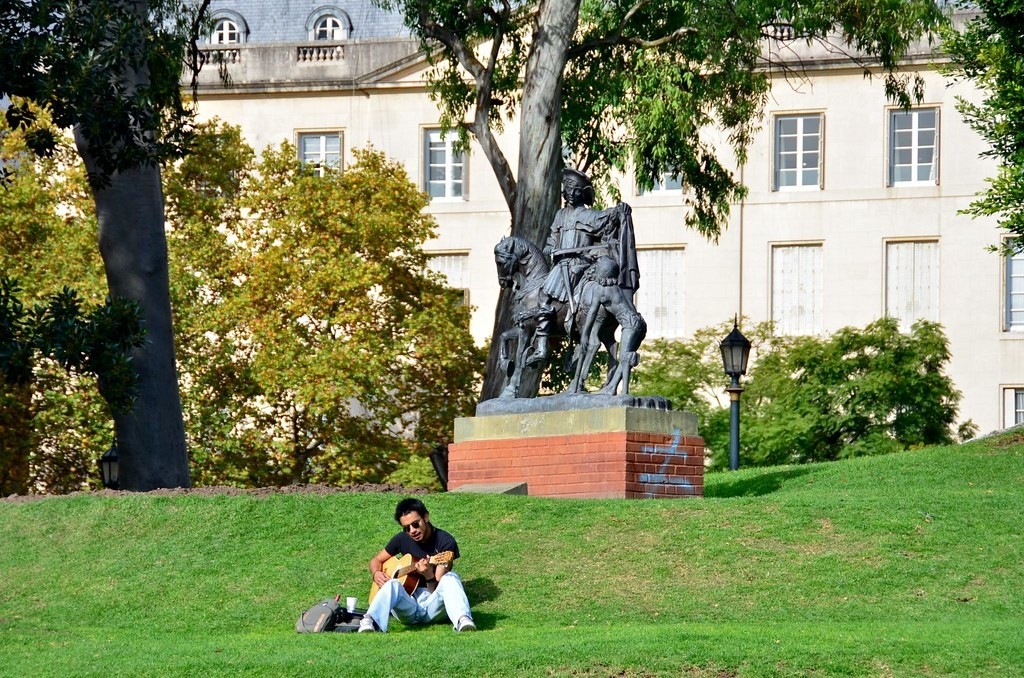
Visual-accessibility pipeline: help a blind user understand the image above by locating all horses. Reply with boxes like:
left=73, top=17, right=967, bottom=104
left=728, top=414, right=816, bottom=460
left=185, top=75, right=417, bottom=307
left=494, top=235, right=619, bottom=398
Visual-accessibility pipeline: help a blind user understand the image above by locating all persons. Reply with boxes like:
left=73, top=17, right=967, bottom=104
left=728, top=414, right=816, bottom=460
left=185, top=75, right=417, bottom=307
left=526, top=169, right=632, bottom=367
left=358, top=498, right=476, bottom=633
left=579, top=257, right=647, bottom=395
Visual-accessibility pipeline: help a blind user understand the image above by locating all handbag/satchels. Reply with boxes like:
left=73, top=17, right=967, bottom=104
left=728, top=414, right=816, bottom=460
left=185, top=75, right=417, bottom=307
left=295, top=598, right=340, bottom=633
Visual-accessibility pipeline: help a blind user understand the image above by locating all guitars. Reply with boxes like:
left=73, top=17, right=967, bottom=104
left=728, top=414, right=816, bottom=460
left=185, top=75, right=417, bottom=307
left=368, top=550, right=455, bottom=606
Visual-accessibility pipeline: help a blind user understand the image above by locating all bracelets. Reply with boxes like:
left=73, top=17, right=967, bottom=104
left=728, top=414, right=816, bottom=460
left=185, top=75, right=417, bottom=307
left=426, top=577, right=436, bottom=583
left=373, top=570, right=380, bottom=582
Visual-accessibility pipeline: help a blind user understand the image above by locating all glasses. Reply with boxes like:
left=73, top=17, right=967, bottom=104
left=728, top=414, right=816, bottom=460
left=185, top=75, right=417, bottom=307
left=402, top=517, right=422, bottom=533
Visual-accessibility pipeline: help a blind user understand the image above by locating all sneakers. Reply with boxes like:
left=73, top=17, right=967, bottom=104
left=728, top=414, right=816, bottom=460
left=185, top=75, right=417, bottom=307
left=358, top=614, right=375, bottom=633
left=459, top=615, right=476, bottom=631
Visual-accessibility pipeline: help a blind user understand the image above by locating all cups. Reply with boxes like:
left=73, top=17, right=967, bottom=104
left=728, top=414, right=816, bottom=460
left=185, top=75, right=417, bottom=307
left=347, top=597, right=358, bottom=613
